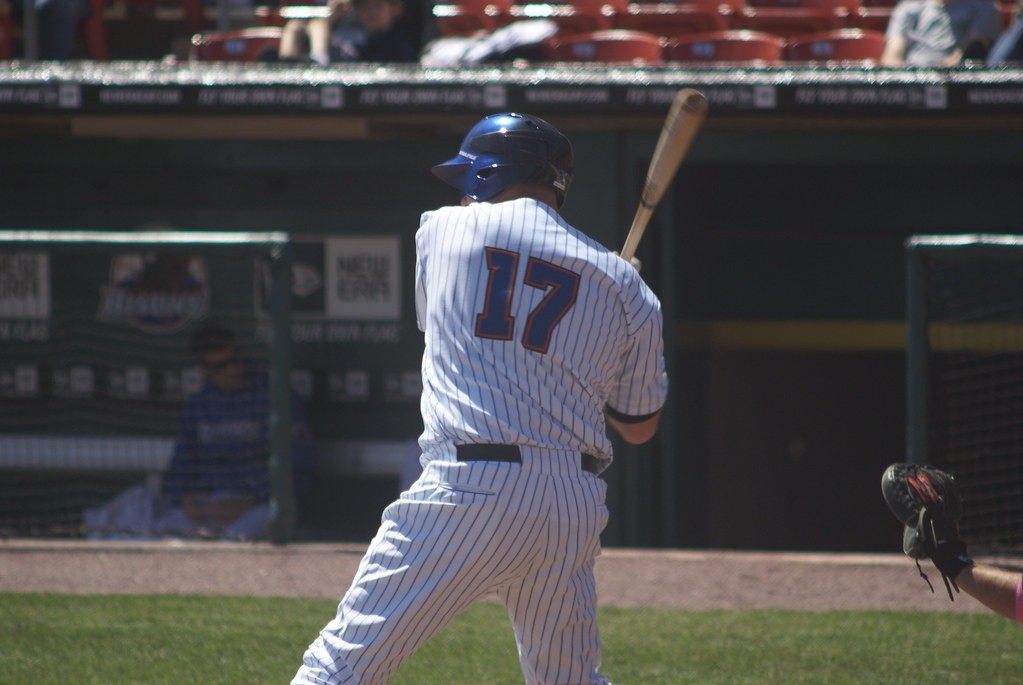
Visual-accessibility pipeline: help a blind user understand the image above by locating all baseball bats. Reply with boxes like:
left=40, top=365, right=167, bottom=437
left=619, top=89, right=708, bottom=262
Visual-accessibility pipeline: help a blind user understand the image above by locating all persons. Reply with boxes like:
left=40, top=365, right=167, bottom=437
left=280, top=0, right=426, bottom=64
left=881, top=464, right=1023, bottom=622
left=151, top=326, right=309, bottom=537
left=881, top=0, right=1005, bottom=67
left=289, top=115, right=670, bottom=685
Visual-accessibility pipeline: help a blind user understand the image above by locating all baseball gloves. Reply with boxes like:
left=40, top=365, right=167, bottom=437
left=883, top=459, right=975, bottom=601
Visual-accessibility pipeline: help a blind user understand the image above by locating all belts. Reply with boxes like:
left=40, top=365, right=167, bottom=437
left=456, top=443, right=597, bottom=475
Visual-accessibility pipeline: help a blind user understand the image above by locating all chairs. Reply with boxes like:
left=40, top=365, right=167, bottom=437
left=0, top=0, right=1023, bottom=69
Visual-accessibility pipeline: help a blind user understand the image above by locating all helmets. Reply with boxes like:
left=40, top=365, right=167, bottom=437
left=431, top=111, right=572, bottom=208
